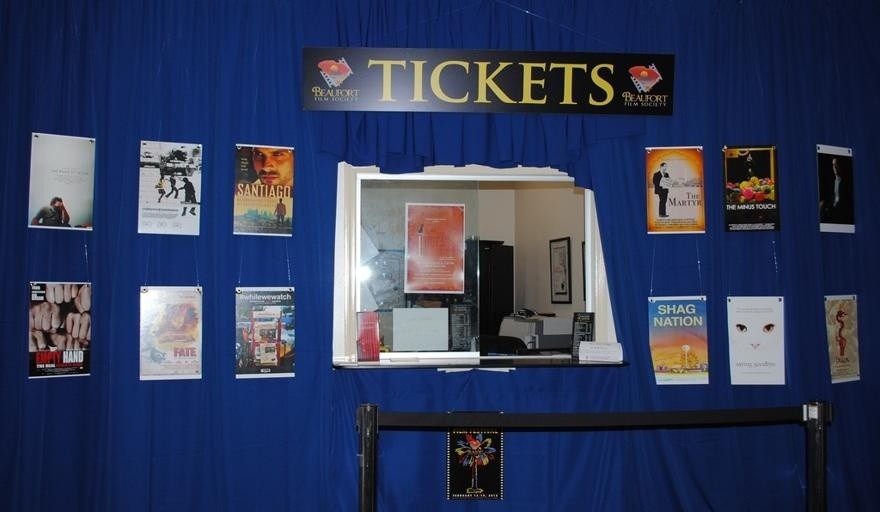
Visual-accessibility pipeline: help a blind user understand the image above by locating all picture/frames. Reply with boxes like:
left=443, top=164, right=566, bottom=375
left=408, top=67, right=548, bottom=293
left=549, top=236, right=572, bottom=304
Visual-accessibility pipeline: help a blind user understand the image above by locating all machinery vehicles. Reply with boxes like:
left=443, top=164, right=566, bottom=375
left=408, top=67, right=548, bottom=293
left=140, top=151, right=160, bottom=168
left=160, top=148, right=197, bottom=176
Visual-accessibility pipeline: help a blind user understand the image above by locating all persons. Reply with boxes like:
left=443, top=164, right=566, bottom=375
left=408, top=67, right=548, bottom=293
left=273, top=197, right=287, bottom=229
left=157, top=176, right=166, bottom=203
left=165, top=174, right=178, bottom=199
left=178, top=177, right=196, bottom=216
left=280, top=305, right=295, bottom=332
left=31, top=197, right=70, bottom=226
left=29, top=283, right=92, bottom=351
left=820, top=156, right=849, bottom=217
left=252, top=147, right=294, bottom=186
left=652, top=161, right=670, bottom=217
